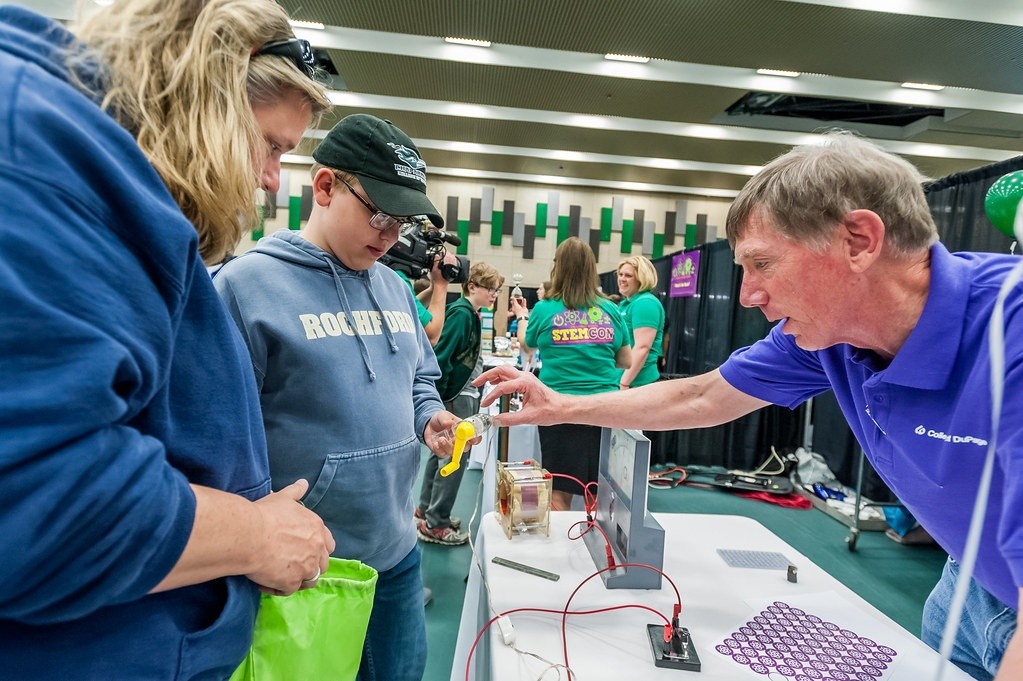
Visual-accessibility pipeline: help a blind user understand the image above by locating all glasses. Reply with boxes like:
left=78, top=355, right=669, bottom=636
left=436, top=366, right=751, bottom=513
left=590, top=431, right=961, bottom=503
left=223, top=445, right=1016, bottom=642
left=474, top=283, right=503, bottom=297
left=252, top=37, right=317, bottom=81
left=332, top=172, right=422, bottom=237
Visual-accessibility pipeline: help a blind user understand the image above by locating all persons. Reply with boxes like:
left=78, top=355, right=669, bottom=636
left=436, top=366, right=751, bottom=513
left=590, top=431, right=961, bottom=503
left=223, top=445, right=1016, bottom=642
left=394, top=237, right=670, bottom=605
left=210, top=113, right=482, bottom=681
left=0, top=0, right=338, bottom=681
left=471, top=124, right=1023, bottom=681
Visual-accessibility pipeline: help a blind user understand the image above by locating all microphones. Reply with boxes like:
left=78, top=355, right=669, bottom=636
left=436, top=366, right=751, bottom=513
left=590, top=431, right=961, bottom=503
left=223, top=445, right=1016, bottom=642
left=442, top=234, right=461, bottom=247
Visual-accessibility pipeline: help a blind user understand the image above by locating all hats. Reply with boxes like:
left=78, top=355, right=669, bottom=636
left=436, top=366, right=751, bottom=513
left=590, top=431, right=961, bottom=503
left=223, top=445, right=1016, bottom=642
left=312, top=114, right=444, bottom=230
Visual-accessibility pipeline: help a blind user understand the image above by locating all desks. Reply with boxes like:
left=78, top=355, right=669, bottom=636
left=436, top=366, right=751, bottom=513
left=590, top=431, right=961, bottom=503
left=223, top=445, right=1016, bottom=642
left=466, top=380, right=585, bottom=521
left=450, top=510, right=978, bottom=681
left=482, top=354, right=542, bottom=369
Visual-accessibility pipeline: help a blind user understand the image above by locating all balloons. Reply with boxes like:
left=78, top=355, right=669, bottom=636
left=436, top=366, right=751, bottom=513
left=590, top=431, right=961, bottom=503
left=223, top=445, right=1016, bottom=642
left=983, top=169, right=1023, bottom=253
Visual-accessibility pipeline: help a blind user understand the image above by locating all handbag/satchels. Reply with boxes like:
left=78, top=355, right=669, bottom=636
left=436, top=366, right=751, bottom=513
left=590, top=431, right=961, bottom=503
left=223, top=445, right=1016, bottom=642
left=227, top=559, right=377, bottom=681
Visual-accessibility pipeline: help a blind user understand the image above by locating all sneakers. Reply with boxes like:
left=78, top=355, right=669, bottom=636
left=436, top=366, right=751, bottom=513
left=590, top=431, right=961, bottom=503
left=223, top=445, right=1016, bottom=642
left=414, top=507, right=462, bottom=528
left=417, top=520, right=469, bottom=544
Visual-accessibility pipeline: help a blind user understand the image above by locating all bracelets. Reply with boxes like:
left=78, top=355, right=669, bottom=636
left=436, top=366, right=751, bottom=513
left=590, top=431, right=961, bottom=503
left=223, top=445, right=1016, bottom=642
left=516, top=316, right=529, bottom=323
